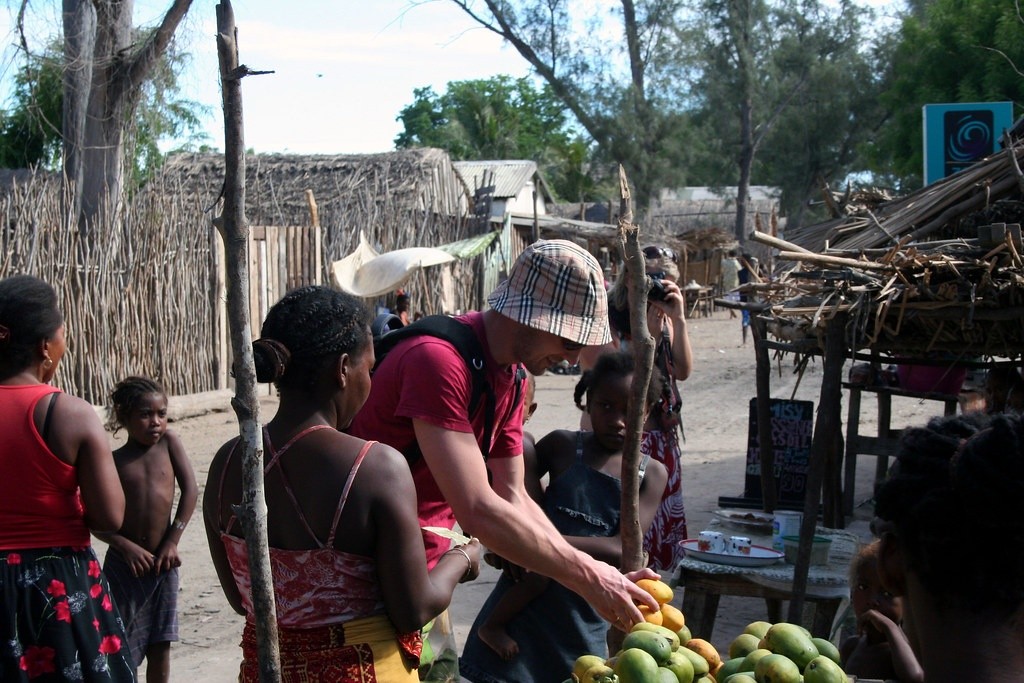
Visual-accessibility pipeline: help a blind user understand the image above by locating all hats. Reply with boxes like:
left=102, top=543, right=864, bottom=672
left=486, top=239, right=613, bottom=346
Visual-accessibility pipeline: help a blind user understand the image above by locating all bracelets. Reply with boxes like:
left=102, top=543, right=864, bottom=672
left=436, top=548, right=472, bottom=582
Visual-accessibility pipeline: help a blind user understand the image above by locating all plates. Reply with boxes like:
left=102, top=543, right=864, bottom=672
left=678, top=539, right=785, bottom=566
left=712, top=508, right=775, bottom=531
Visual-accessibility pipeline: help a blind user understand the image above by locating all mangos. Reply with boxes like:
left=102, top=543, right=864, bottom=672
left=559, top=578, right=852, bottom=683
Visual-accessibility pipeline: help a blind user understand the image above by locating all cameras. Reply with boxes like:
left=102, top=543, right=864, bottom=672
left=645, top=271, right=674, bottom=305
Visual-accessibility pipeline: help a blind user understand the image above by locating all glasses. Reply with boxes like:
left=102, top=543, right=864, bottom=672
left=562, top=337, right=586, bottom=350
left=643, top=245, right=674, bottom=260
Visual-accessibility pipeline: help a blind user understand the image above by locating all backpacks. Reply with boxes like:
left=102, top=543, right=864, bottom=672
left=368, top=313, right=527, bottom=463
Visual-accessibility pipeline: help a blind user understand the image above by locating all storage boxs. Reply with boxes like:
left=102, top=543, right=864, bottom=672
left=780, top=534, right=834, bottom=567
left=898, top=366, right=967, bottom=397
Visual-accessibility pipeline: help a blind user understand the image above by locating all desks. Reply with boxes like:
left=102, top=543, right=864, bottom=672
left=668, top=508, right=861, bottom=644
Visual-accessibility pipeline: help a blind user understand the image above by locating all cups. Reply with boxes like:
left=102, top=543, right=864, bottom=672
left=726, top=536, right=751, bottom=556
left=699, top=531, right=727, bottom=554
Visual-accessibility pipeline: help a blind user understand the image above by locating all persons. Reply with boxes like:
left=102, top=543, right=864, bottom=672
left=343, top=242, right=663, bottom=683
left=0, top=274, right=141, bottom=683
left=735, top=254, right=763, bottom=349
left=578, top=244, right=694, bottom=573
left=388, top=294, right=410, bottom=319
left=457, top=349, right=670, bottom=683
left=202, top=284, right=483, bottom=682
left=87, top=372, right=202, bottom=683
left=838, top=363, right=1024, bottom=683
left=718, top=250, right=742, bottom=320
